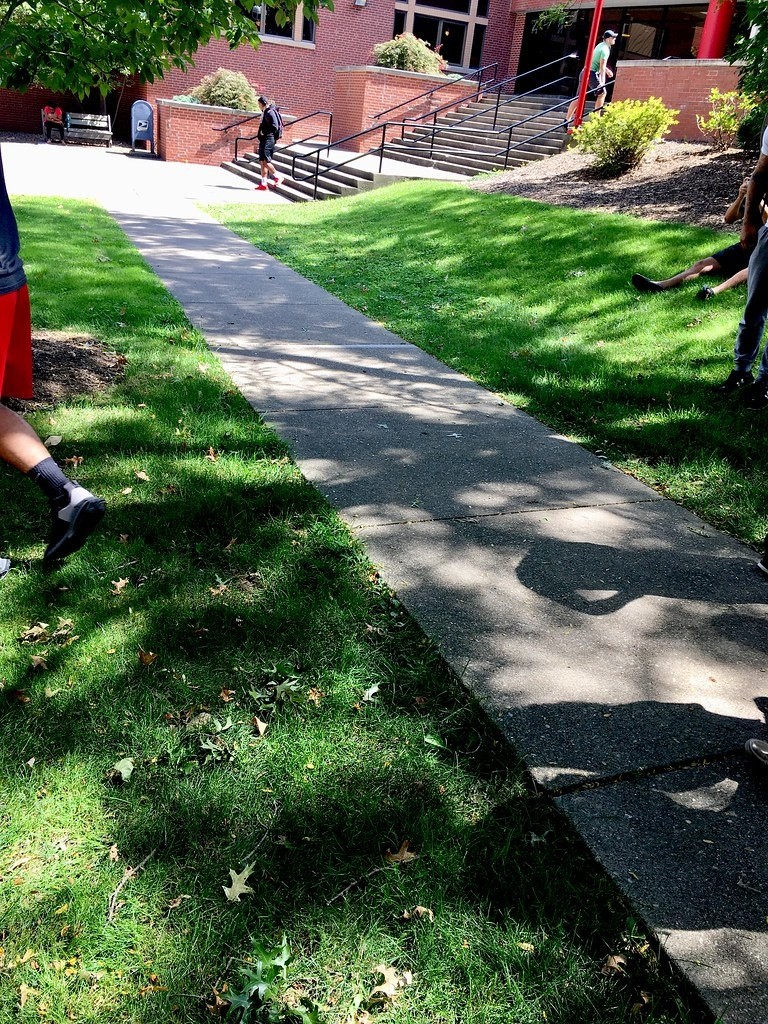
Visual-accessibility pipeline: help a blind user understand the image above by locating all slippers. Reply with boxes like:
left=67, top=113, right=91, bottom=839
left=703, top=285, right=714, bottom=301
left=632, top=273, right=663, bottom=293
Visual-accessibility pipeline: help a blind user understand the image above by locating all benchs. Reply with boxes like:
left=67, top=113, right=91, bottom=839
left=41, top=110, right=113, bottom=148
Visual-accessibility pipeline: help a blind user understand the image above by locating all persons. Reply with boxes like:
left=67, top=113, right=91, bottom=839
left=632, top=177, right=768, bottom=300
left=561, top=30, right=619, bottom=134
left=43, top=99, right=66, bottom=145
left=254, top=96, right=285, bottom=191
left=0, top=145, right=106, bottom=560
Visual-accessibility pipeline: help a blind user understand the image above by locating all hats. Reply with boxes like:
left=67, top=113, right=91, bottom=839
left=604, top=30, right=619, bottom=39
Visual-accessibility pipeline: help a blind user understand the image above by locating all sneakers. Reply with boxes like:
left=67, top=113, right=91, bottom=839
left=0, top=557, right=11, bottom=580
left=746, top=383, right=768, bottom=411
left=719, top=369, right=756, bottom=393
left=44, top=480, right=108, bottom=561
left=255, top=184, right=269, bottom=191
left=273, top=176, right=284, bottom=189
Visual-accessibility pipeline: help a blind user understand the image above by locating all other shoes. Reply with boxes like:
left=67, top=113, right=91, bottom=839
left=561, top=122, right=569, bottom=133
left=744, top=738, right=768, bottom=769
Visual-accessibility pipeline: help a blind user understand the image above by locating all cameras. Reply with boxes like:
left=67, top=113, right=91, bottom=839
left=747, top=178, right=750, bottom=185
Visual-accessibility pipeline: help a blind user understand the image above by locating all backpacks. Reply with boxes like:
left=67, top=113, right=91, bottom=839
left=267, top=107, right=283, bottom=140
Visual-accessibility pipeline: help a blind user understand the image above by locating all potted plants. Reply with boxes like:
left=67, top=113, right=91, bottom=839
left=332, top=31, right=489, bottom=156
left=155, top=66, right=297, bottom=167
